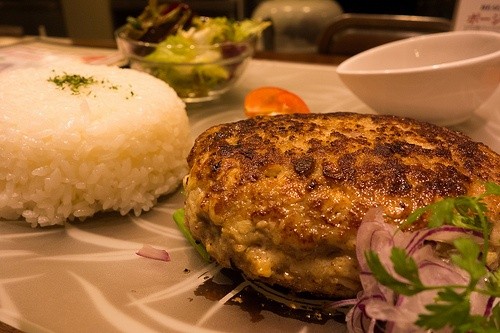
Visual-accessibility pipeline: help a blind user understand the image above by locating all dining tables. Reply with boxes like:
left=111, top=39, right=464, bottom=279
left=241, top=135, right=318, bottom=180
left=1, top=38, right=500, bottom=333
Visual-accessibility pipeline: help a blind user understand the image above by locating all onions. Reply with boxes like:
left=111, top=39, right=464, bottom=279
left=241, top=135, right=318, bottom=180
left=326, top=182, right=499, bottom=333
left=135, top=245, right=170, bottom=262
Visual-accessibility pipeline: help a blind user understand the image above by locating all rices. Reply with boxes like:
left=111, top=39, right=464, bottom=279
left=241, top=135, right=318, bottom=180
left=1, top=65, right=194, bottom=228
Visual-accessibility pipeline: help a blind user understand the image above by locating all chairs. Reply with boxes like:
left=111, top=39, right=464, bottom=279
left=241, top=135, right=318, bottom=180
left=317, top=15, right=452, bottom=59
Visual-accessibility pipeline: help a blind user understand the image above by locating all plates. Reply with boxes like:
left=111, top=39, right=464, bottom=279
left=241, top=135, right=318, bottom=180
left=208, top=239, right=372, bottom=314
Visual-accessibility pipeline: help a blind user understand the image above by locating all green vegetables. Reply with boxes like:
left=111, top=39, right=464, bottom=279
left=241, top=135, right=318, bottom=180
left=116, top=0, right=273, bottom=100
left=171, top=208, right=212, bottom=264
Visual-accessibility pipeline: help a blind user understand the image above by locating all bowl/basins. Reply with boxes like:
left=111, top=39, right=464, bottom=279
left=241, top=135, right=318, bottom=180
left=338, top=27, right=500, bottom=131
left=113, top=10, right=253, bottom=104
left=249, top=0, right=343, bottom=55
left=330, top=11, right=452, bottom=54
